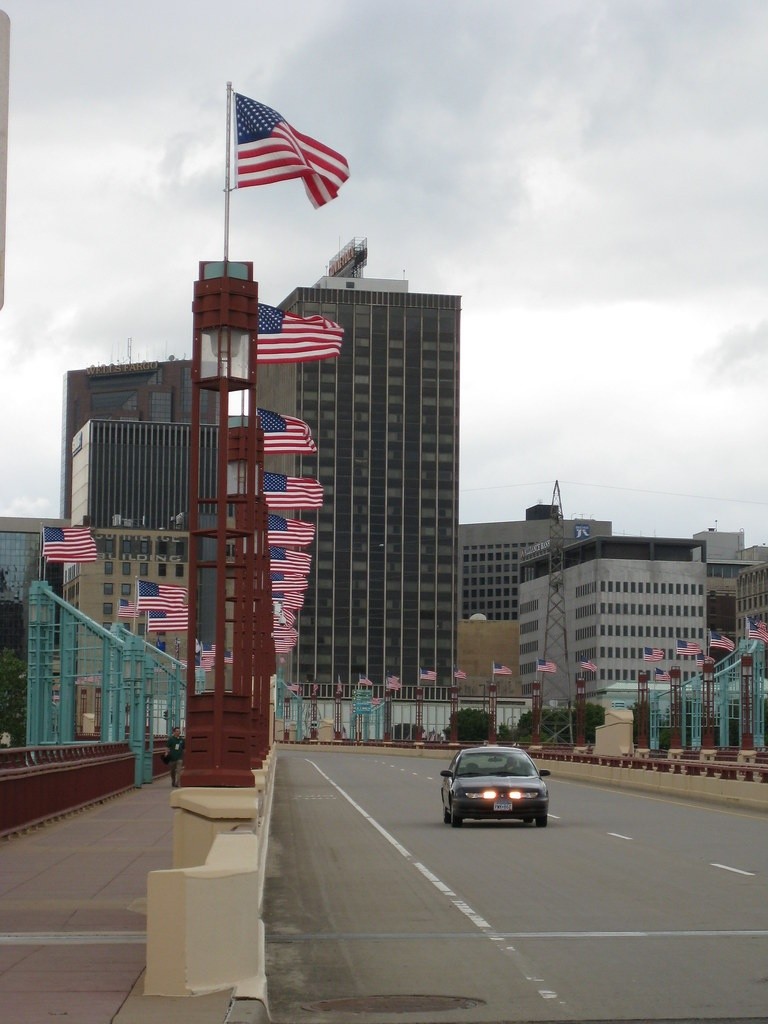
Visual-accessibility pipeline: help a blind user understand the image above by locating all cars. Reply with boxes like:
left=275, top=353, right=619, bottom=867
left=439, top=747, right=551, bottom=828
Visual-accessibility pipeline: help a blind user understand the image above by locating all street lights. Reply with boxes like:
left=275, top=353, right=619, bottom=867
left=478, top=683, right=486, bottom=712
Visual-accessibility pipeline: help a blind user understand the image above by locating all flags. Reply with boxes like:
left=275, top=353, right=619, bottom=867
left=194, top=654, right=216, bottom=672
left=136, top=580, right=188, bottom=611
left=263, top=472, right=324, bottom=512
left=43, top=526, right=92, bottom=556
left=747, top=618, right=768, bottom=642
left=388, top=673, right=401, bottom=689
left=172, top=659, right=186, bottom=669
left=270, top=571, right=309, bottom=654
left=257, top=408, right=317, bottom=454
left=287, top=682, right=303, bottom=691
left=645, top=648, right=665, bottom=662
left=267, top=514, right=315, bottom=547
left=421, top=670, right=436, bottom=680
left=147, top=604, right=189, bottom=632
left=232, top=91, right=351, bottom=210
left=118, top=598, right=139, bottom=618
left=269, top=546, right=313, bottom=575
left=224, top=652, right=234, bottom=663
left=655, top=668, right=671, bottom=681
left=359, top=675, right=373, bottom=686
left=258, top=302, right=346, bottom=363
left=581, top=656, right=598, bottom=672
left=48, top=535, right=98, bottom=562
left=538, top=659, right=557, bottom=673
left=677, top=631, right=735, bottom=667
left=201, top=644, right=216, bottom=657
left=453, top=668, right=466, bottom=678
left=494, top=663, right=513, bottom=674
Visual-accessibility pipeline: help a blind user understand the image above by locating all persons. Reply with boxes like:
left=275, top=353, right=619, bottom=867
left=167, top=727, right=185, bottom=787
left=506, top=756, right=526, bottom=774
